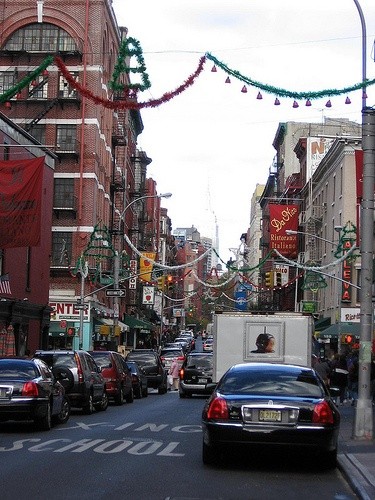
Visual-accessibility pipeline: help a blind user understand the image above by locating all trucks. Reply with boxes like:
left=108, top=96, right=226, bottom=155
left=213, top=312, right=314, bottom=397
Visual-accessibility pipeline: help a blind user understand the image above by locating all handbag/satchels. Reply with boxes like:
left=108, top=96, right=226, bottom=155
left=167, top=375, right=173, bottom=385
left=329, top=387, right=340, bottom=396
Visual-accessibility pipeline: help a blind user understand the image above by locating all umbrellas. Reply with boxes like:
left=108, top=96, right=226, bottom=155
left=4, top=322, right=16, bottom=356
left=0, top=325, right=8, bottom=359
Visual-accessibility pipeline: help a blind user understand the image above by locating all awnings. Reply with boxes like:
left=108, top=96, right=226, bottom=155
left=48, top=321, right=75, bottom=337
left=320, top=322, right=360, bottom=339
left=101, top=318, right=128, bottom=331
left=125, top=314, right=153, bottom=328
left=93, top=319, right=112, bottom=341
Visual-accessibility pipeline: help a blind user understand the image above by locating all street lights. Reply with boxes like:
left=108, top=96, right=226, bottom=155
left=284, top=229, right=350, bottom=358
left=112, top=189, right=172, bottom=351
left=75, top=264, right=166, bottom=347
left=271, top=261, right=375, bottom=300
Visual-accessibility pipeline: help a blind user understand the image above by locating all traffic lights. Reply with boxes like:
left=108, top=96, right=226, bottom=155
left=341, top=333, right=355, bottom=343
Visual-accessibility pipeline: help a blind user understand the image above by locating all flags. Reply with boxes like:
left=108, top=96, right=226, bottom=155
left=0, top=273, right=11, bottom=294
left=269, top=203, right=299, bottom=256
left=0, top=156, right=45, bottom=247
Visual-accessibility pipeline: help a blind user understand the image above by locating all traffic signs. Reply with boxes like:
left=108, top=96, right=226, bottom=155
left=105, top=289, right=126, bottom=297
left=73, top=304, right=90, bottom=311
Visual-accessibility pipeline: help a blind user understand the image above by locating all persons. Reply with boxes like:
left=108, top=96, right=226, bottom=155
left=312, top=350, right=359, bottom=406
left=167, top=357, right=179, bottom=391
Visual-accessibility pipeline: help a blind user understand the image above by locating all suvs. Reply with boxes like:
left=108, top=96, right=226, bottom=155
left=177, top=353, right=218, bottom=399
left=124, top=346, right=168, bottom=395
left=34, top=348, right=110, bottom=413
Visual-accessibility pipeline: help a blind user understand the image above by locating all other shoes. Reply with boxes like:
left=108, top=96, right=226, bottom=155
left=167, top=388, right=171, bottom=391
left=340, top=403, right=343, bottom=406
left=175, top=389, right=178, bottom=391
left=333, top=400, right=336, bottom=403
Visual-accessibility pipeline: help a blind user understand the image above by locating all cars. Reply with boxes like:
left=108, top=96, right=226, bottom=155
left=201, top=362, right=341, bottom=471
left=0, top=356, right=71, bottom=435
left=125, top=360, right=146, bottom=401
left=81, top=349, right=133, bottom=405
left=164, top=323, right=213, bottom=352
